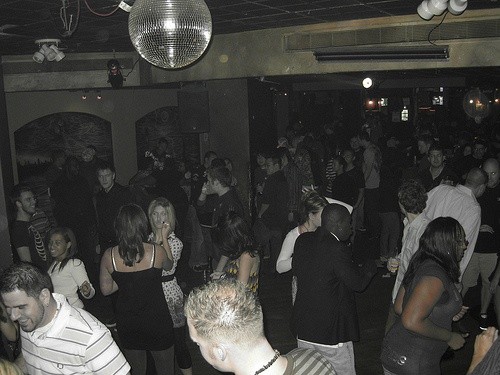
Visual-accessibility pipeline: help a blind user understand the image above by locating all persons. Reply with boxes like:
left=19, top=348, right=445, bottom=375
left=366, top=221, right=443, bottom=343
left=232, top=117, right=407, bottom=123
left=0, top=121, right=500, bottom=375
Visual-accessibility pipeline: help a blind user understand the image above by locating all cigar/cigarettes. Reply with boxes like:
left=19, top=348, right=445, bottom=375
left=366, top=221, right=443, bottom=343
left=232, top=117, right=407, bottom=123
left=480, top=326, right=488, bottom=331
left=202, top=187, right=207, bottom=191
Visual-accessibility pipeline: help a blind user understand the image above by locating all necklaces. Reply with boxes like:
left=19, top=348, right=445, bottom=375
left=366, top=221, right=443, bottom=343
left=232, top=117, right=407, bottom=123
left=303, top=224, right=310, bottom=233
left=254, top=349, right=280, bottom=375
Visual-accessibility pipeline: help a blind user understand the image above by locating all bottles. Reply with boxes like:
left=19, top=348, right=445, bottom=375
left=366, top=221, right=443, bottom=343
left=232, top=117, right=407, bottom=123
left=390, top=248, right=399, bottom=275
left=400, top=105, right=410, bottom=122
left=206, top=255, right=213, bottom=282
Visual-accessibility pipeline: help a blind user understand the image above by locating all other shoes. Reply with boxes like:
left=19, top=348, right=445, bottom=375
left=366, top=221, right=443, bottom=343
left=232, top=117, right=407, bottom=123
left=453, top=306, right=469, bottom=323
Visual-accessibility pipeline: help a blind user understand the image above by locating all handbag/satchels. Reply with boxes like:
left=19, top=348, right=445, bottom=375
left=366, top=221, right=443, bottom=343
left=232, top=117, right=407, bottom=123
left=440, top=314, right=492, bottom=375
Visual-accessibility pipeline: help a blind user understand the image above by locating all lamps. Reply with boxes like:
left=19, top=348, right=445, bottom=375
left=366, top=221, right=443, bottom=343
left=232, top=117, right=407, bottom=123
left=31, top=38, right=65, bottom=64
left=313, top=47, right=450, bottom=62
left=127, top=0, right=213, bottom=69
left=417, top=0, right=467, bottom=20
